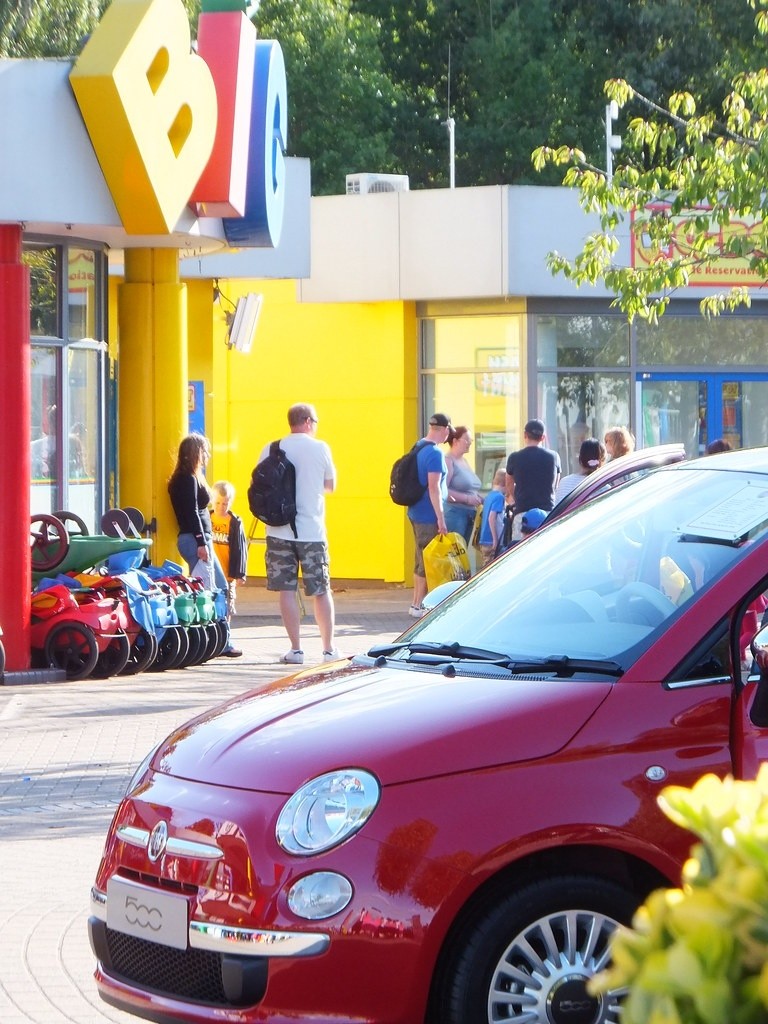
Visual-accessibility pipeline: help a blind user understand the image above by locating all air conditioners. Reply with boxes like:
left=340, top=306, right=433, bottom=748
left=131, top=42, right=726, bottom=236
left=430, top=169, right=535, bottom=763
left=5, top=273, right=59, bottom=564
left=346, top=173, right=409, bottom=194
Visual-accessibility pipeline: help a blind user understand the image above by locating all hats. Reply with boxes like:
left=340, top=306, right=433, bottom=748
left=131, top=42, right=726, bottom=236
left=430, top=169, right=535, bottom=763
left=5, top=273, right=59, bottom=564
left=521, top=508, right=548, bottom=529
left=526, top=419, right=544, bottom=435
left=429, top=413, right=451, bottom=428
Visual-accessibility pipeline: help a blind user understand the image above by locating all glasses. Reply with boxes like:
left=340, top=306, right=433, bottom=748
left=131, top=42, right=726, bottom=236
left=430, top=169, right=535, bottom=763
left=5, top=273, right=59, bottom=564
left=456, top=439, right=474, bottom=443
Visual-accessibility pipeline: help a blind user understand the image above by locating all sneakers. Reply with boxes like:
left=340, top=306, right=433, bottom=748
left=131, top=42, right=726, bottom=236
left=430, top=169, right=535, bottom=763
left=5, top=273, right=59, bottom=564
left=324, top=649, right=345, bottom=663
left=409, top=606, right=430, bottom=618
left=279, top=650, right=304, bottom=664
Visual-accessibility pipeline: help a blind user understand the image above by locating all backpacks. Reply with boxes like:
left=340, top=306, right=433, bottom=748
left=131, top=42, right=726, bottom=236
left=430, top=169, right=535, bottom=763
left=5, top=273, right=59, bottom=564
left=248, top=439, right=296, bottom=527
left=390, top=442, right=436, bottom=506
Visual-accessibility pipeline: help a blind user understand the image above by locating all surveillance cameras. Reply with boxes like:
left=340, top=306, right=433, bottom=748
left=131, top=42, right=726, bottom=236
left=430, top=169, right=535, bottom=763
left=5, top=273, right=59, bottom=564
left=228, top=292, right=263, bottom=354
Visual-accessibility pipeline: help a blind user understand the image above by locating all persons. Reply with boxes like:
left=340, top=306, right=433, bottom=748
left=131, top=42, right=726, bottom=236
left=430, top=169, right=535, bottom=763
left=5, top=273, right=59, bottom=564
left=444, top=426, right=485, bottom=549
left=29, top=407, right=89, bottom=479
left=406, top=413, right=456, bottom=618
left=168, top=434, right=243, bottom=658
left=479, top=469, right=507, bottom=565
left=520, top=508, right=548, bottom=539
left=209, top=482, right=247, bottom=622
left=258, top=403, right=342, bottom=664
left=740, top=593, right=768, bottom=670
left=703, top=440, right=732, bottom=583
left=604, top=427, right=646, bottom=577
left=505, top=419, right=562, bottom=542
left=555, top=438, right=642, bottom=577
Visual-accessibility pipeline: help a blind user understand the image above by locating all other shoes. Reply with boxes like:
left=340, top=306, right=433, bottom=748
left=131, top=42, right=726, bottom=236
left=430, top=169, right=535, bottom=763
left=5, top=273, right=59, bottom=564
left=221, top=649, right=241, bottom=657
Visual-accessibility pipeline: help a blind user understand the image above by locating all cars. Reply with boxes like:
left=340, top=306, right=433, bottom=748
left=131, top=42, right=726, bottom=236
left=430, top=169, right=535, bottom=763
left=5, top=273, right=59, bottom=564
left=88, top=441, right=768, bottom=1024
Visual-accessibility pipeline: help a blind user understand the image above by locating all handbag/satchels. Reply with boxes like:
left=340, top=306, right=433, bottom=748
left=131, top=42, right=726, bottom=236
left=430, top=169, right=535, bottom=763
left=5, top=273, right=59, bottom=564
left=192, top=558, right=216, bottom=591
left=423, top=533, right=471, bottom=592
